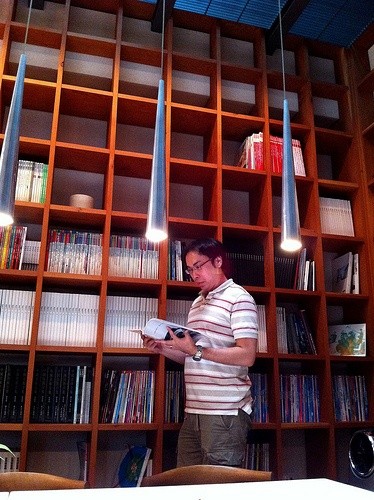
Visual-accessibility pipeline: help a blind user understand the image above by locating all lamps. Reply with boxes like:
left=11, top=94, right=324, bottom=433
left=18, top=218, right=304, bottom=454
left=0, top=1, right=32, bottom=226
left=145, top=0, right=168, bottom=242
left=280, top=0, right=304, bottom=251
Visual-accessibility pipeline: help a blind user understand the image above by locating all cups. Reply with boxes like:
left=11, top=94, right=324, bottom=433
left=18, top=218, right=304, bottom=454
left=70, top=194, right=93, bottom=209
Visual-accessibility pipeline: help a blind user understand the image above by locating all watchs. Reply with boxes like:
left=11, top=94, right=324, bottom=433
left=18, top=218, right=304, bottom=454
left=193, top=345, right=202, bottom=361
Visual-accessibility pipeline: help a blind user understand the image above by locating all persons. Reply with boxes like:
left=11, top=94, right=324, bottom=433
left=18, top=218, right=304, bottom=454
left=128, top=318, right=206, bottom=345
left=175, top=239, right=257, bottom=469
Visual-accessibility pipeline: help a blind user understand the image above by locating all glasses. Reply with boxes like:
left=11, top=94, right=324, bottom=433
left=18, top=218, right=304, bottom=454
left=185, top=259, right=211, bottom=274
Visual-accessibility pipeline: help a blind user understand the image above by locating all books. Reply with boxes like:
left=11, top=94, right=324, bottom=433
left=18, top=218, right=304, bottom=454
left=332, top=252, right=353, bottom=293
left=166, top=300, right=193, bottom=327
left=46, top=229, right=103, bottom=275
left=256, top=305, right=268, bottom=353
left=0, top=226, right=28, bottom=271
left=168, top=239, right=194, bottom=282
left=274, top=247, right=316, bottom=291
left=319, top=197, right=355, bottom=237
left=103, top=295, right=159, bottom=349
left=76, top=440, right=271, bottom=487
left=224, top=252, right=265, bottom=287
left=0, top=364, right=369, bottom=424
left=0, top=289, right=36, bottom=345
left=108, top=234, right=159, bottom=280
left=37, top=292, right=99, bottom=347
left=270, top=135, right=282, bottom=174
left=235, top=132, right=263, bottom=170
left=351, top=254, right=360, bottom=294
left=22, top=241, right=41, bottom=271
left=14, top=159, right=50, bottom=204
left=292, top=139, right=307, bottom=177
left=276, top=305, right=318, bottom=356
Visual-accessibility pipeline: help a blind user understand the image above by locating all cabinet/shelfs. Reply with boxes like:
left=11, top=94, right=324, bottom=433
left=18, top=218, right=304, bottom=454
left=0, top=0, right=374, bottom=490
